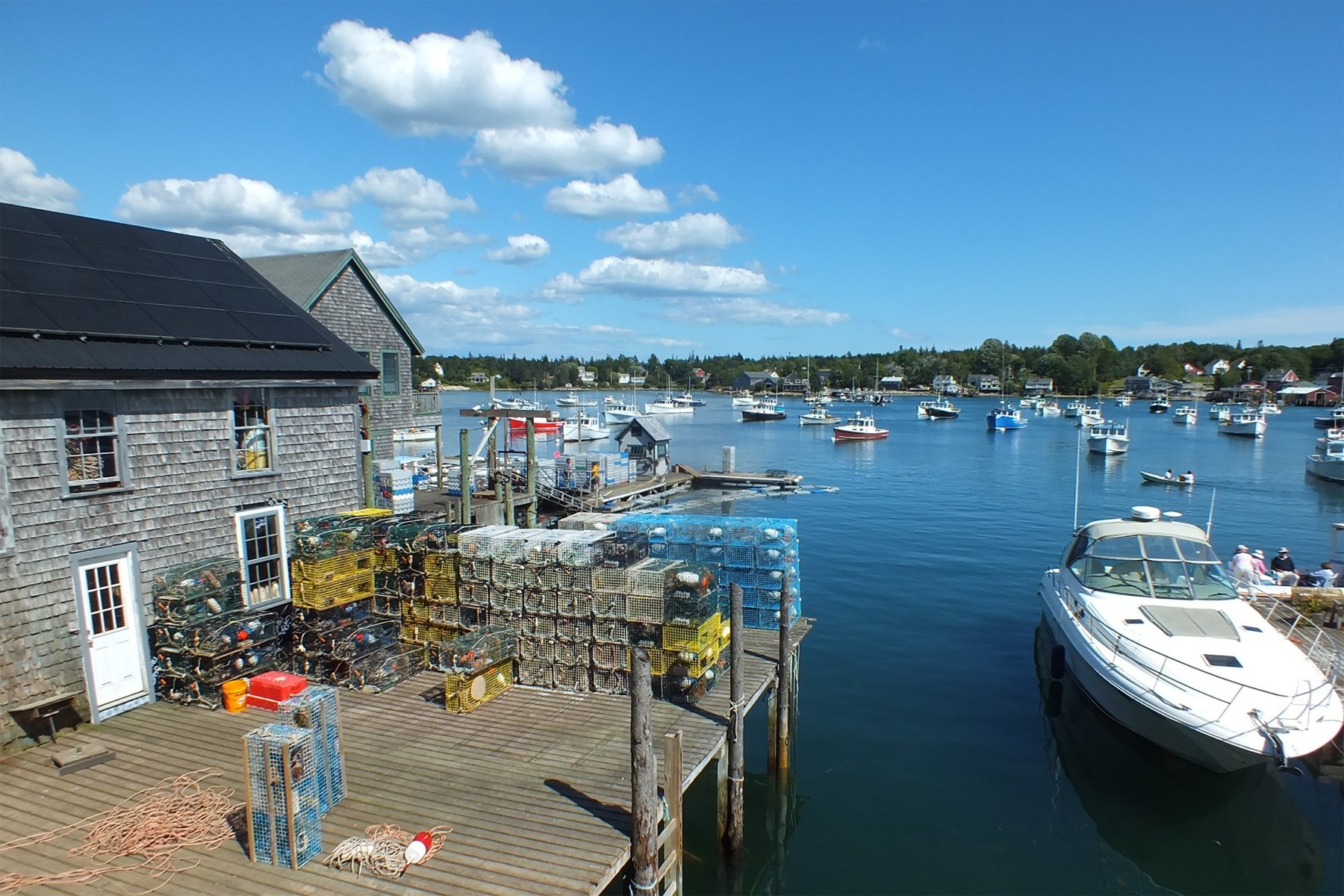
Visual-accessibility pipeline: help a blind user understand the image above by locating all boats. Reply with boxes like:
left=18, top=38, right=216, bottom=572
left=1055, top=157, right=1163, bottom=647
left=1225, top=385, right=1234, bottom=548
left=1172, top=390, right=1198, bottom=425
left=1208, top=382, right=1282, bottom=420
left=1032, top=613, right=1327, bottom=896
left=1036, top=430, right=1343, bottom=775
left=1115, top=395, right=1131, bottom=407
left=1149, top=384, right=1171, bottom=414
left=732, top=393, right=761, bottom=406
left=833, top=411, right=890, bottom=440
left=798, top=404, right=841, bottom=425
left=1217, top=401, right=1268, bottom=439
left=740, top=403, right=787, bottom=421
left=804, top=355, right=893, bottom=406
left=1018, top=378, right=1105, bottom=427
left=917, top=393, right=961, bottom=420
left=1140, top=470, right=1194, bottom=486
left=1306, top=375, right=1344, bottom=481
left=1087, top=418, right=1130, bottom=456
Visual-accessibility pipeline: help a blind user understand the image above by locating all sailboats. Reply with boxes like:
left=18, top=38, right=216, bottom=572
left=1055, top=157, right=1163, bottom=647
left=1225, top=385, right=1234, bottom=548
left=753, top=382, right=786, bottom=411
left=986, top=347, right=1028, bottom=429
left=499, top=370, right=708, bottom=442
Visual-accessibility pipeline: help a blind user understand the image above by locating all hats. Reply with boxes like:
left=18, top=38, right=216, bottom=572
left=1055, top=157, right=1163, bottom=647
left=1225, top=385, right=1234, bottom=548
left=1278, top=547, right=1288, bottom=553
left=1251, top=550, right=1264, bottom=559
left=1237, top=545, right=1248, bottom=550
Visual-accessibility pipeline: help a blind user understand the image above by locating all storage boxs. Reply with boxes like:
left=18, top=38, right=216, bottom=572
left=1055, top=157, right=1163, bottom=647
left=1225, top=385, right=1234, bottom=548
left=246, top=671, right=307, bottom=712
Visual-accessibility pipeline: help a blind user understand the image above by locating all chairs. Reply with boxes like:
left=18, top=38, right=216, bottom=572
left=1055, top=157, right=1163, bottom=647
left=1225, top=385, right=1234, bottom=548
left=1083, top=560, right=1165, bottom=578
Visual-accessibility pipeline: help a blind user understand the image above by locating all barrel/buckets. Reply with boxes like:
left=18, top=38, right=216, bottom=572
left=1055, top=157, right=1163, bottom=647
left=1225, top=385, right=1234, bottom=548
left=245, top=451, right=267, bottom=469
left=222, top=680, right=247, bottom=713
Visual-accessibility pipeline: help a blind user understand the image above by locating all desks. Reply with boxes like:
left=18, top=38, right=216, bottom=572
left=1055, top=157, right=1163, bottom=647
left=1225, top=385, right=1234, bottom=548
left=8, top=690, right=86, bottom=744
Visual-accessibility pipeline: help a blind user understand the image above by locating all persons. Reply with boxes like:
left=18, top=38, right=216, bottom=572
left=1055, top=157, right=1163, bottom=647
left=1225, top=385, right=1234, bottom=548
left=587, top=463, right=602, bottom=490
left=563, top=458, right=573, bottom=487
left=1230, top=545, right=1335, bottom=600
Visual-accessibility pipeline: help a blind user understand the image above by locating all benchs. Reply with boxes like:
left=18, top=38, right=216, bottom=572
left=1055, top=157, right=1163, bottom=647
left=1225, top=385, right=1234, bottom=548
left=1266, top=573, right=1324, bottom=588
